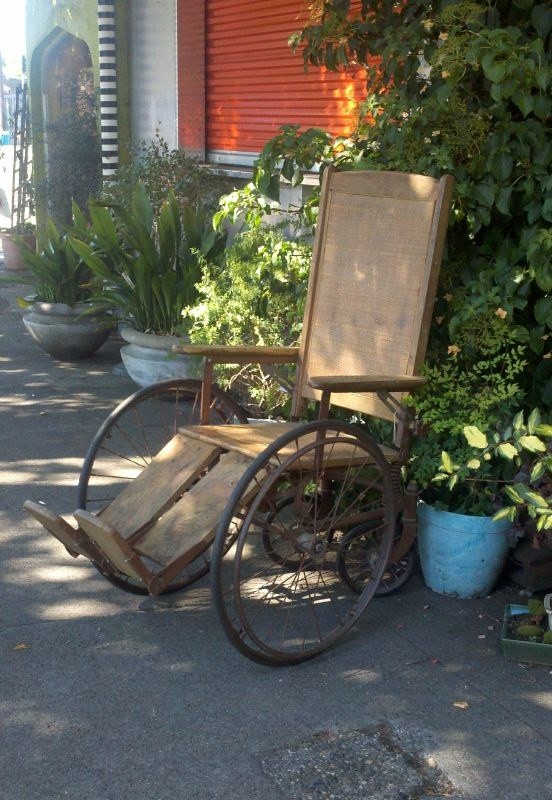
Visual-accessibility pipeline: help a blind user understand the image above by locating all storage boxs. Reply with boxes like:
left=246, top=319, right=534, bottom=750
left=501, top=600, right=552, bottom=666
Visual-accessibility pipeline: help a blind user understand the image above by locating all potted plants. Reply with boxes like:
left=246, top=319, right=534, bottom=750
left=67, top=172, right=229, bottom=403
left=414, top=309, right=517, bottom=600
left=0, top=222, right=39, bottom=273
left=10, top=215, right=114, bottom=362
